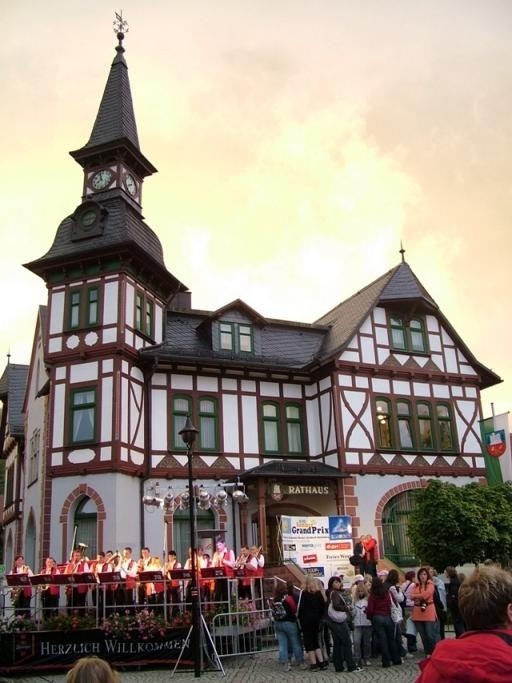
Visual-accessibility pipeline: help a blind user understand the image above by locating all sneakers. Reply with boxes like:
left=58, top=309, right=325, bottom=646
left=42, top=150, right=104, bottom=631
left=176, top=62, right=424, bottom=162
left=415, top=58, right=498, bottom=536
left=283, top=652, right=414, bottom=672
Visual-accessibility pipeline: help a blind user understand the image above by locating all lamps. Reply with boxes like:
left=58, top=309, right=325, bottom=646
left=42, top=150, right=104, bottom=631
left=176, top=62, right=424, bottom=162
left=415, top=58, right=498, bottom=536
left=142, top=481, right=249, bottom=513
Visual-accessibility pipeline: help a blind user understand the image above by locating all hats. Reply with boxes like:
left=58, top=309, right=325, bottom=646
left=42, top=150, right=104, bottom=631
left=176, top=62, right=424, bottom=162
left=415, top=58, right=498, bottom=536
left=352, top=574, right=364, bottom=584
left=376, top=569, right=389, bottom=576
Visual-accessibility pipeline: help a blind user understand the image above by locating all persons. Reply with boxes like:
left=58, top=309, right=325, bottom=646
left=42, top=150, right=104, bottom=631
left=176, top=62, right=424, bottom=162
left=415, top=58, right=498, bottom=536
left=362, top=534, right=379, bottom=577
left=63, top=656, right=122, bottom=683
left=353, top=535, right=368, bottom=577
left=408, top=564, right=512, bottom=683
left=267, top=565, right=467, bottom=675
left=332, top=518, right=348, bottom=532
left=2, top=538, right=266, bottom=626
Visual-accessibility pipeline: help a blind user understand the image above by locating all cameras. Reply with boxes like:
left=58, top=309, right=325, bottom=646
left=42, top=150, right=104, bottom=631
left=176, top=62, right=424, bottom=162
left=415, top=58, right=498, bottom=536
left=421, top=599, right=427, bottom=612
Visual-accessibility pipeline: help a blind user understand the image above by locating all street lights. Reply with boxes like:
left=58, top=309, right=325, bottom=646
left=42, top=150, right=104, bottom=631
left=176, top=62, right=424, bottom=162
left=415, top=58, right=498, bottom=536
left=177, top=412, right=208, bottom=678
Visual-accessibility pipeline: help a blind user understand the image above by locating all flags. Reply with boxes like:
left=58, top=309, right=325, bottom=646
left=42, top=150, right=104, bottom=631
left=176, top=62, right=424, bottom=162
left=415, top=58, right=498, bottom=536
left=478, top=410, right=511, bottom=486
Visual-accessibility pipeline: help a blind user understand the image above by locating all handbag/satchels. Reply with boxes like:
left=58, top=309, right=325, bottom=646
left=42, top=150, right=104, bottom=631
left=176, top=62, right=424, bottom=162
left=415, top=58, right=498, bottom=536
left=390, top=604, right=403, bottom=624
left=327, top=601, right=348, bottom=623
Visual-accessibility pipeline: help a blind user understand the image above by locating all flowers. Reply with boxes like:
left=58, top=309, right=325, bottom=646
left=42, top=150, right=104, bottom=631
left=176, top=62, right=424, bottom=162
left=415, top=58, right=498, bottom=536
left=0, top=597, right=259, bottom=639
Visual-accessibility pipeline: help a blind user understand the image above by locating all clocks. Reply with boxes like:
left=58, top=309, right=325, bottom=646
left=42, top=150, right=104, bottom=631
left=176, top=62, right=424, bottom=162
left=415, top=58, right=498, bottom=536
left=87, top=168, right=116, bottom=192
left=123, top=169, right=139, bottom=199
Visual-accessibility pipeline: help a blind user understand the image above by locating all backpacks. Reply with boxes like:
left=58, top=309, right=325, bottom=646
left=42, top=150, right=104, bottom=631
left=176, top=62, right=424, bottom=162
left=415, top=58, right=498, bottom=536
left=269, top=595, right=288, bottom=622
